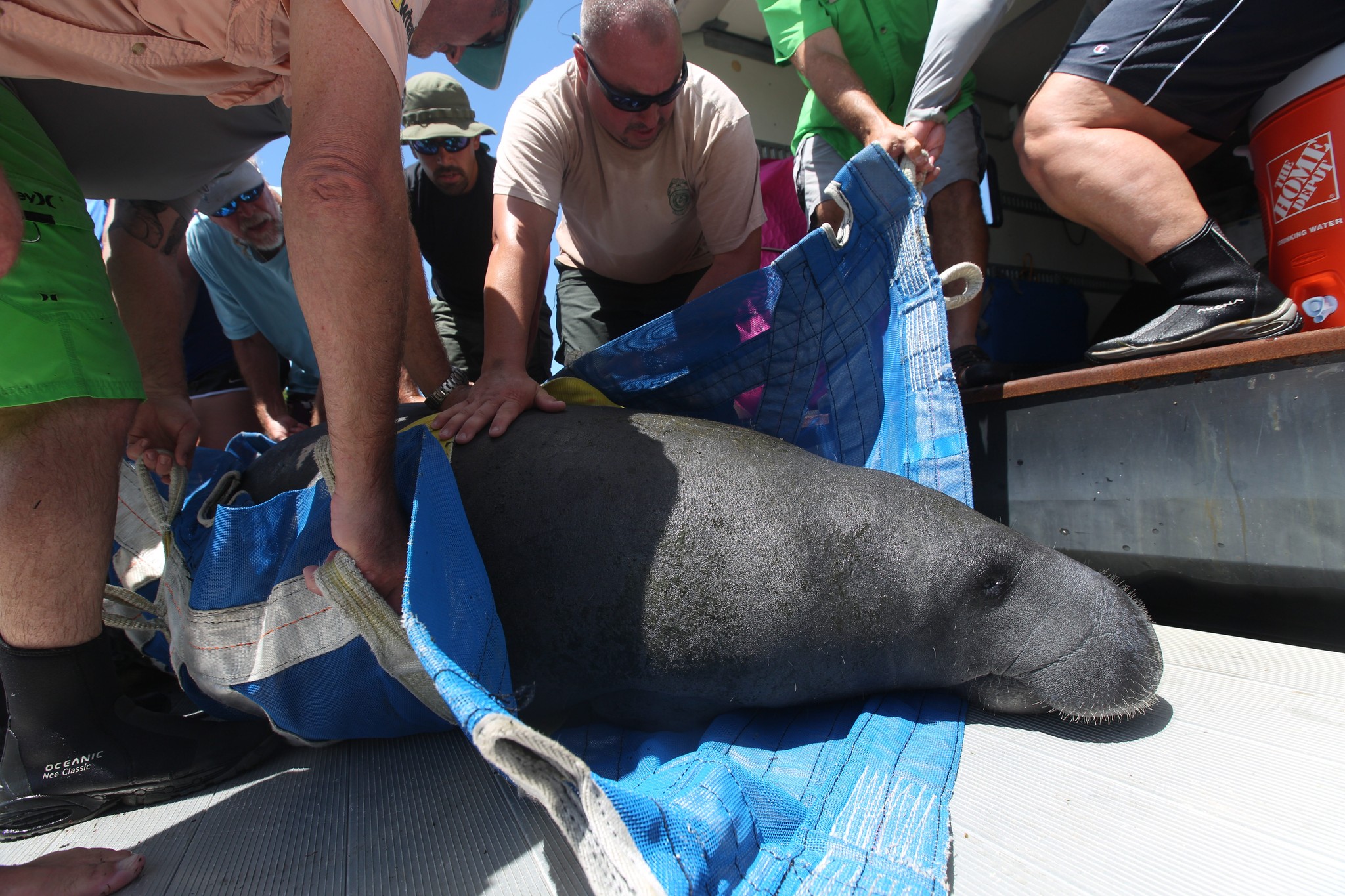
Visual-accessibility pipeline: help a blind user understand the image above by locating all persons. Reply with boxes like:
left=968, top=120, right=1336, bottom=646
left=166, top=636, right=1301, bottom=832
left=0, top=0, right=539, bottom=896
left=758, top=0, right=1020, bottom=392
left=428, top=0, right=767, bottom=445
left=901, top=0, right=1345, bottom=367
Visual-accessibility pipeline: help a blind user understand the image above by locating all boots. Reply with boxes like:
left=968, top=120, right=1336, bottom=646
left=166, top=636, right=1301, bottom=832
left=1098, top=146, right=1274, bottom=337
left=0, top=626, right=292, bottom=843
left=1091, top=215, right=1301, bottom=358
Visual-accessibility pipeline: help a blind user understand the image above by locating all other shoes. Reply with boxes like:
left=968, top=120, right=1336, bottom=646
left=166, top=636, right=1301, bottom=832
left=954, top=346, right=1014, bottom=385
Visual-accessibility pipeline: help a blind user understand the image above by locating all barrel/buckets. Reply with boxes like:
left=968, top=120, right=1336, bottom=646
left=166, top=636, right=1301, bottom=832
left=1243, top=43, right=1345, bottom=334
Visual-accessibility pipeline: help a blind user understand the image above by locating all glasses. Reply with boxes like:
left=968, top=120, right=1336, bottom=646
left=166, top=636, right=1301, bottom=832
left=467, top=0, right=512, bottom=48
left=211, top=181, right=264, bottom=217
left=570, top=33, right=688, bottom=112
left=409, top=137, right=471, bottom=155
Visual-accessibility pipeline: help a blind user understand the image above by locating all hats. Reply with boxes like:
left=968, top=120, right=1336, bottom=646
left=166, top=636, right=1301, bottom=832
left=181, top=158, right=262, bottom=216
left=400, top=71, right=497, bottom=140
left=453, top=0, right=532, bottom=90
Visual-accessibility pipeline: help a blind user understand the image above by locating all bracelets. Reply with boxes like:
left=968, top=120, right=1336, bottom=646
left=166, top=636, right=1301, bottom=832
left=423, top=368, right=469, bottom=414
